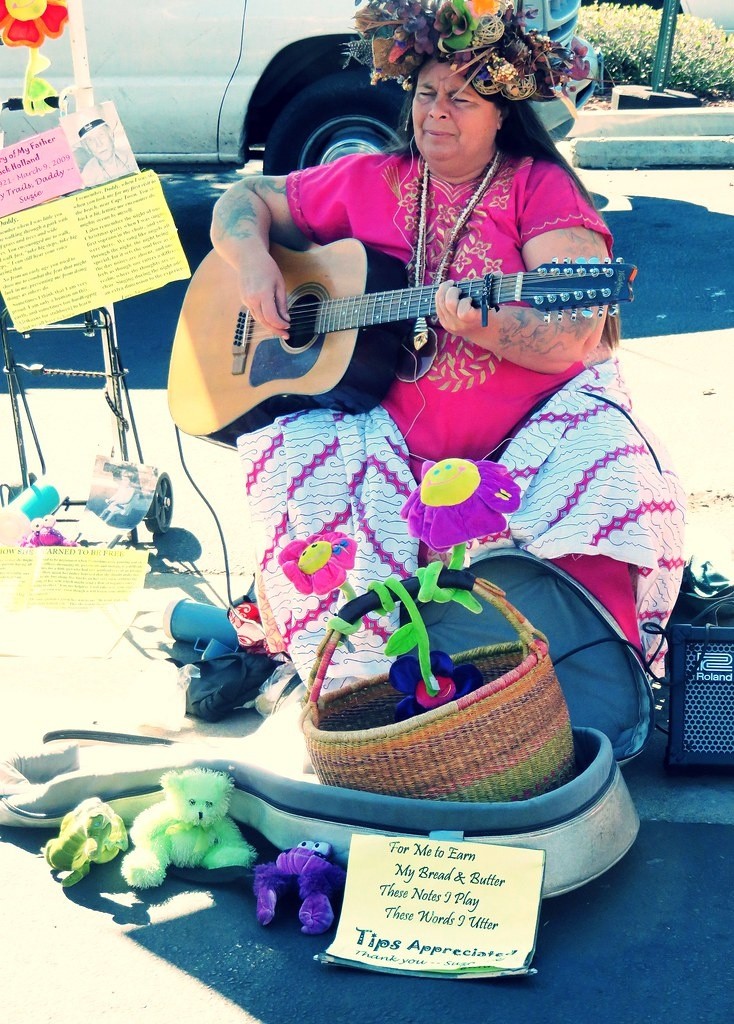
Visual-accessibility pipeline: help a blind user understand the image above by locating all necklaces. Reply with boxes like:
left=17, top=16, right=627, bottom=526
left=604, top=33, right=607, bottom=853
left=407, top=148, right=503, bottom=348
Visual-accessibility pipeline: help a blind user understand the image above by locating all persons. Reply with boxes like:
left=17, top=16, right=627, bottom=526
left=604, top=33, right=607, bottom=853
left=210, top=52, right=643, bottom=698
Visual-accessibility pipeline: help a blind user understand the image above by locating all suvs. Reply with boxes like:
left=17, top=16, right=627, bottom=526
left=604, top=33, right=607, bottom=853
left=0, top=0, right=597, bottom=205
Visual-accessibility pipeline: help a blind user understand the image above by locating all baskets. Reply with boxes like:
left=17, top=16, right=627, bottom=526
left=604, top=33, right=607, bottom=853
left=296, top=568, right=581, bottom=805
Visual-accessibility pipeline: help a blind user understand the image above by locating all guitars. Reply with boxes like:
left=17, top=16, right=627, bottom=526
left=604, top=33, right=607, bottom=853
left=164, top=235, right=641, bottom=445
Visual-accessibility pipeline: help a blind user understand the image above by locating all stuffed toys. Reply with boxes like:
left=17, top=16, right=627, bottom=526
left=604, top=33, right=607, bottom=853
left=122, top=767, right=256, bottom=888
left=45, top=797, right=129, bottom=888
left=252, top=839, right=348, bottom=934
